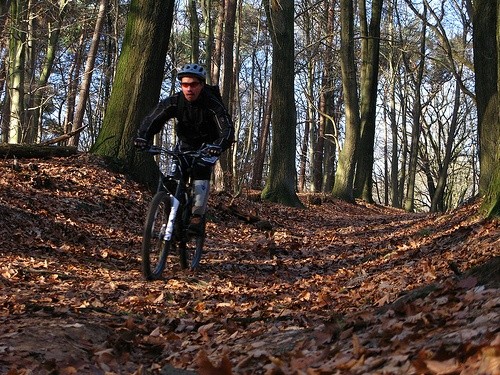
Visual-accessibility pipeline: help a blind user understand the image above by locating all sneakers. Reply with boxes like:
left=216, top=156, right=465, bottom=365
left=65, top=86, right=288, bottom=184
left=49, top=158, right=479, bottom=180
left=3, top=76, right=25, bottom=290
left=185, top=217, right=202, bottom=234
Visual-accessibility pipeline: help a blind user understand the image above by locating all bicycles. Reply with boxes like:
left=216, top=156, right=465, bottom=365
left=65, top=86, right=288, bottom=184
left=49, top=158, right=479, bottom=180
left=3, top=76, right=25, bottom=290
left=129, top=136, right=218, bottom=281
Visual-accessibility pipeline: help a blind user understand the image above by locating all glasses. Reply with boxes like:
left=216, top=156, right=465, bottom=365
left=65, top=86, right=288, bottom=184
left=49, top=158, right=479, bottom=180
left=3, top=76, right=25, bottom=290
left=179, top=80, right=203, bottom=88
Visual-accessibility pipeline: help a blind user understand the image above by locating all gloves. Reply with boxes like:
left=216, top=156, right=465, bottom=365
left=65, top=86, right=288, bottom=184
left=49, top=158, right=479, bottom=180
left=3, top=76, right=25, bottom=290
left=206, top=144, right=222, bottom=157
left=134, top=137, right=148, bottom=147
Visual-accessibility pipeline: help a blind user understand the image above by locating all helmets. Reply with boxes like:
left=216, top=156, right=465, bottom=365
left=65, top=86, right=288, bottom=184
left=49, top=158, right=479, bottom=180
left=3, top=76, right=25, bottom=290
left=177, top=64, right=206, bottom=79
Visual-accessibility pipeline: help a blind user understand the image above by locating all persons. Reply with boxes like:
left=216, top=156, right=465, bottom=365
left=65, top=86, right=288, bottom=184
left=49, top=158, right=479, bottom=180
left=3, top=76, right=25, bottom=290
left=132, top=64, right=236, bottom=238
